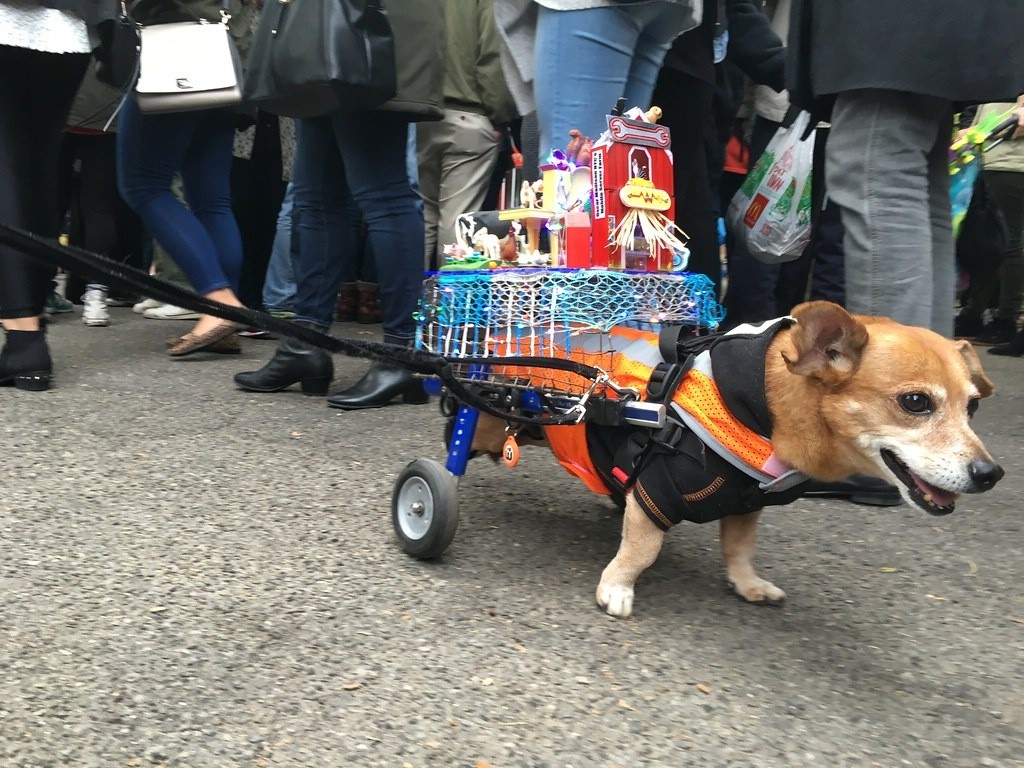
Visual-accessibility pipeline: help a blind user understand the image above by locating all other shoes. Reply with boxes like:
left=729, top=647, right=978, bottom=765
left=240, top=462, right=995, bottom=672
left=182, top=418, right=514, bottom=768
left=802, top=472, right=902, bottom=506
left=131, top=299, right=203, bottom=320
left=163, top=304, right=248, bottom=356
left=105, top=297, right=133, bottom=307
left=954, top=302, right=1024, bottom=357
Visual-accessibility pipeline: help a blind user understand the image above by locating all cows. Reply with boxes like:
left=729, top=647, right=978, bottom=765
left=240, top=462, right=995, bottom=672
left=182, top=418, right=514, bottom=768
left=455, top=208, right=550, bottom=257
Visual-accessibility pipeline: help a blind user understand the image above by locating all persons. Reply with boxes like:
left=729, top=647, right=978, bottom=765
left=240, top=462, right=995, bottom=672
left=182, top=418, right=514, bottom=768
left=0, top=0, right=1024, bottom=507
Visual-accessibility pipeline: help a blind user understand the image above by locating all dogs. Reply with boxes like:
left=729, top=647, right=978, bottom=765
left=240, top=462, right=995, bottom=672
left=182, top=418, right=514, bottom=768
left=447, top=300, right=1005, bottom=619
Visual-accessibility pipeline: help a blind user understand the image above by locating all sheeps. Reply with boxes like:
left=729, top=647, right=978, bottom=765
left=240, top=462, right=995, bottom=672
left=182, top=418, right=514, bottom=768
left=520, top=178, right=544, bottom=208
left=473, top=226, right=501, bottom=261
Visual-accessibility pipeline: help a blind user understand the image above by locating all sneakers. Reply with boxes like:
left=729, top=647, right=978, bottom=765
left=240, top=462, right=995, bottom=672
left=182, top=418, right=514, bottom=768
left=81, top=290, right=111, bottom=327
left=42, top=280, right=75, bottom=312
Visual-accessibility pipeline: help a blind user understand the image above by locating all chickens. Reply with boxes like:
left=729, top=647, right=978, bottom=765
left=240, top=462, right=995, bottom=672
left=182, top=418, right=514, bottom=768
left=518, top=249, right=551, bottom=266
left=499, top=226, right=516, bottom=266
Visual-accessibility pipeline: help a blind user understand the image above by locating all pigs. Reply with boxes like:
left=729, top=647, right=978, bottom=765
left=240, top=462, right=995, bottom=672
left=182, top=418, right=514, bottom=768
left=442, top=242, right=466, bottom=259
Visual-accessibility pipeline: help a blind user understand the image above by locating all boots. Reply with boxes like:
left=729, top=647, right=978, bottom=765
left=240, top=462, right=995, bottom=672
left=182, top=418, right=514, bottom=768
left=0, top=330, right=52, bottom=391
left=327, top=329, right=429, bottom=409
left=233, top=319, right=334, bottom=396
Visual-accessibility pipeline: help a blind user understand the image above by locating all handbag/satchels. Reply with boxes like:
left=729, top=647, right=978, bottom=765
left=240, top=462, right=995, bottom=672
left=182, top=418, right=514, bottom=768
left=241, top=0, right=397, bottom=120
left=118, top=8, right=245, bottom=116
left=723, top=109, right=817, bottom=265
